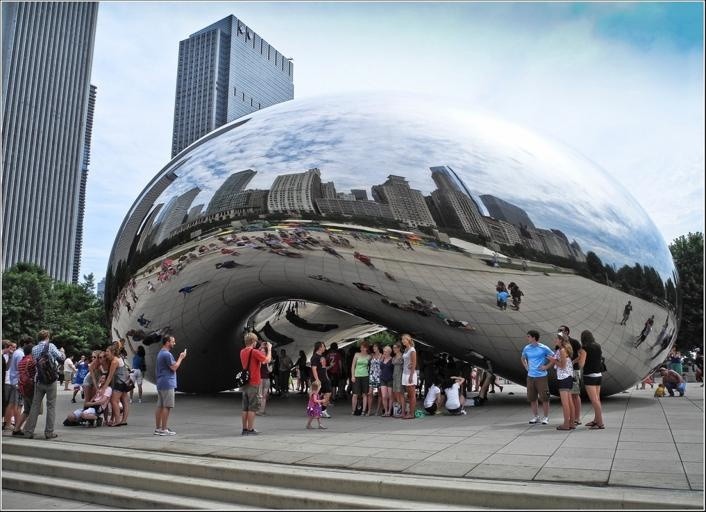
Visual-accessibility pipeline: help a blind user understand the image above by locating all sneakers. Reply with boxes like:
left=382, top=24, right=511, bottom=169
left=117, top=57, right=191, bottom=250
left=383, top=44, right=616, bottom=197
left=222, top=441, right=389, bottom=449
left=12, top=430, right=24, bottom=435
left=154, top=428, right=176, bottom=436
left=242, top=431, right=262, bottom=436
left=25, top=433, right=32, bottom=438
left=541, top=416, right=548, bottom=424
left=46, top=434, right=57, bottom=438
left=529, top=416, right=540, bottom=424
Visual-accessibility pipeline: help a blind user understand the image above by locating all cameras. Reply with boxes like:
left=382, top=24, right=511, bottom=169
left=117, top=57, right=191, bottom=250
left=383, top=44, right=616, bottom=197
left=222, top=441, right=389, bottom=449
left=264, top=342, right=270, bottom=347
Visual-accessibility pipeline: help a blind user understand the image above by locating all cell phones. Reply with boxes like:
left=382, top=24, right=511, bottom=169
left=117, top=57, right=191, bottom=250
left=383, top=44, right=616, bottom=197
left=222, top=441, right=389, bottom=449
left=184, top=349, right=186, bottom=353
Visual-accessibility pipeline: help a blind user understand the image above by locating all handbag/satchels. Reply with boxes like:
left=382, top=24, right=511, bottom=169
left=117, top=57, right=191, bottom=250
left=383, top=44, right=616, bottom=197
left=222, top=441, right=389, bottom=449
left=600, top=357, right=607, bottom=372
left=233, top=369, right=250, bottom=388
left=415, top=408, right=425, bottom=418
left=571, top=381, right=580, bottom=394
left=125, top=378, right=135, bottom=391
left=393, top=401, right=410, bottom=417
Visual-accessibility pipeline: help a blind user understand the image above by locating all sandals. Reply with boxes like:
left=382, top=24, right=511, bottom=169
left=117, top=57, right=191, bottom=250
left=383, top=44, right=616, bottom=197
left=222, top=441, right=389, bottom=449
left=585, top=421, right=605, bottom=429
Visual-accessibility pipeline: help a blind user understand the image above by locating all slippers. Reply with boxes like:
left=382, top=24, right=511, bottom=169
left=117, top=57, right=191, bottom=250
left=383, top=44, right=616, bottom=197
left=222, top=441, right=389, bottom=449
left=557, top=420, right=582, bottom=430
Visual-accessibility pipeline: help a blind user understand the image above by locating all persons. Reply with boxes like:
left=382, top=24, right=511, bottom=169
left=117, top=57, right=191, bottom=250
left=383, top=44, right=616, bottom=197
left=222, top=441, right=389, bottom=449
left=108, top=224, right=478, bottom=361
left=236, top=333, right=272, bottom=437
left=516, top=325, right=703, bottom=433
left=256, top=334, right=502, bottom=419
left=306, top=380, right=328, bottom=429
left=621, top=300, right=633, bottom=324
left=494, top=280, right=525, bottom=311
left=1, top=325, right=146, bottom=441
left=634, top=314, right=675, bottom=360
left=154, top=334, right=186, bottom=437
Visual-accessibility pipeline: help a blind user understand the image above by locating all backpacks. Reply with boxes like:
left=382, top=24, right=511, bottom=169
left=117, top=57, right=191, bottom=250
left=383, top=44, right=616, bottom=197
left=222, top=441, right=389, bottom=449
left=18, top=372, right=33, bottom=397
left=36, top=352, right=59, bottom=385
